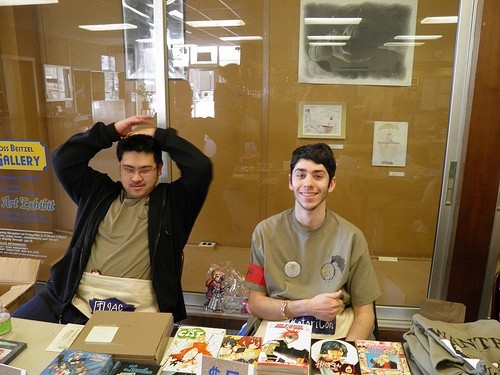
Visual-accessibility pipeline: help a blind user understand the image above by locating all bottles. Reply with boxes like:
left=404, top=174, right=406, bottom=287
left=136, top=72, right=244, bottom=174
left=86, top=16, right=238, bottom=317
left=0, top=304, right=13, bottom=341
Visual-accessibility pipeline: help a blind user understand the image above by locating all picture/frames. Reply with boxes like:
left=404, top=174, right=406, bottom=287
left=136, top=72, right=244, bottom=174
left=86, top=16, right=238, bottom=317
left=297, top=100, right=348, bottom=139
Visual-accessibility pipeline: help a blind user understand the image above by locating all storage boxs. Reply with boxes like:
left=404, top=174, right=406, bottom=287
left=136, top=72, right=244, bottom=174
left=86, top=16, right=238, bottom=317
left=68, top=310, right=173, bottom=366
left=0, top=256, right=41, bottom=314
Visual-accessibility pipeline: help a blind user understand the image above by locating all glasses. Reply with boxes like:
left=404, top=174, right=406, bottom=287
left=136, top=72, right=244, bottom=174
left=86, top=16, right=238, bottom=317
left=120, top=163, right=158, bottom=176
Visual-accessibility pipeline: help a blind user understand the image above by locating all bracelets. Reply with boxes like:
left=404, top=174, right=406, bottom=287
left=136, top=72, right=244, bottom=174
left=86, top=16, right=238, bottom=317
left=281, top=300, right=291, bottom=319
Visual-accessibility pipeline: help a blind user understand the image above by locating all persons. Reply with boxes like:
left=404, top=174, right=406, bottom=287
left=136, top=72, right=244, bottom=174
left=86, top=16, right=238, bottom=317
left=245, top=143, right=382, bottom=341
left=10, top=115, right=213, bottom=336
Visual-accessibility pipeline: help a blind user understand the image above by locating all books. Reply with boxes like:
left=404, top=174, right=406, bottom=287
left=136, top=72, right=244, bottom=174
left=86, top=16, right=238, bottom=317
left=0, top=322, right=411, bottom=375
